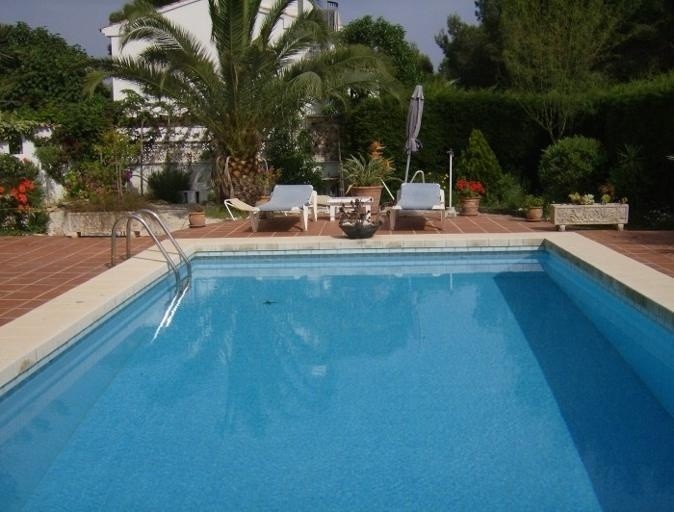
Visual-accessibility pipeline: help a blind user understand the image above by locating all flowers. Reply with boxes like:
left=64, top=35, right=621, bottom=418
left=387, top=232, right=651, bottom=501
left=456, top=178, right=485, bottom=197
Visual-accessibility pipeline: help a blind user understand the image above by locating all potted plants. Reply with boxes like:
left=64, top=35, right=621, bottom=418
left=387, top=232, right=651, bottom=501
left=522, top=194, right=545, bottom=222
left=338, top=152, right=405, bottom=215
left=188, top=204, right=207, bottom=227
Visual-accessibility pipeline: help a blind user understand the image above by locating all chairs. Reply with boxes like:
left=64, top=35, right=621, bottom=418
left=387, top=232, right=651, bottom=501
left=250, top=184, right=317, bottom=233
left=387, top=183, right=447, bottom=230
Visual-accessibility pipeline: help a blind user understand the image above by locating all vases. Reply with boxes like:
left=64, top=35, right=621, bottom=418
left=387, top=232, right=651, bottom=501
left=460, top=197, right=481, bottom=216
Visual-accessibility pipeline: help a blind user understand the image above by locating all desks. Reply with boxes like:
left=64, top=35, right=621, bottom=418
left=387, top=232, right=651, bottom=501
left=327, top=197, right=374, bottom=221
left=550, top=203, right=629, bottom=232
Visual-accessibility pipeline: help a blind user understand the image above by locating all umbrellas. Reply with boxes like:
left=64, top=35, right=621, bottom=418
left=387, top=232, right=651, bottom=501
left=403, top=84, right=425, bottom=183
